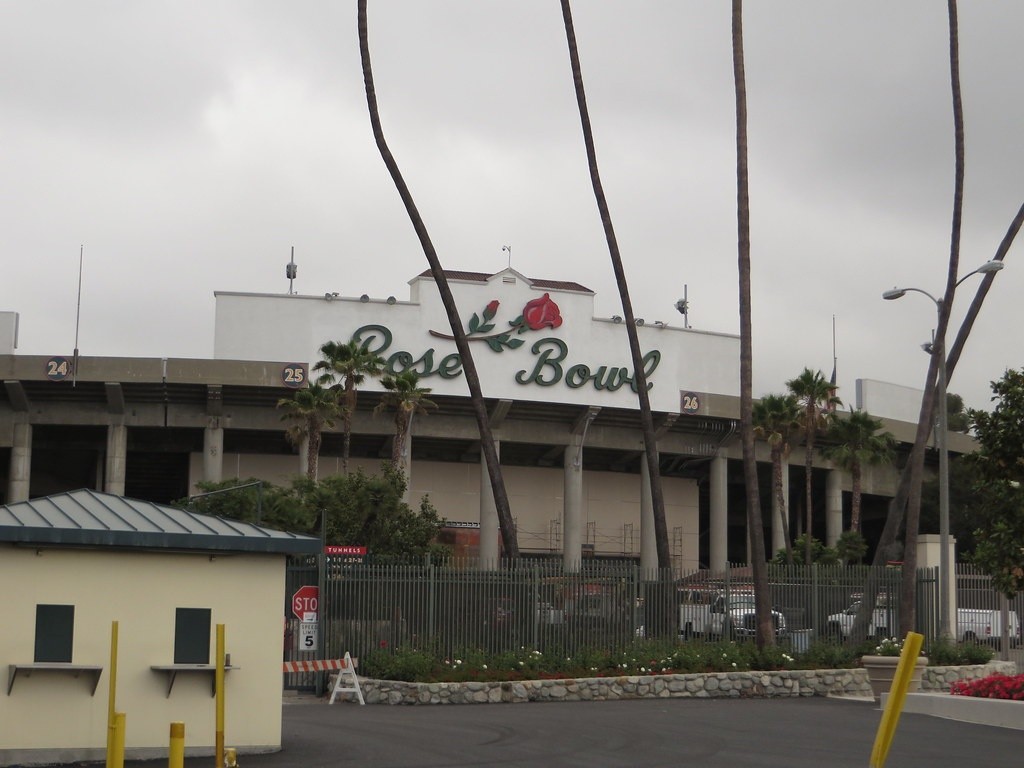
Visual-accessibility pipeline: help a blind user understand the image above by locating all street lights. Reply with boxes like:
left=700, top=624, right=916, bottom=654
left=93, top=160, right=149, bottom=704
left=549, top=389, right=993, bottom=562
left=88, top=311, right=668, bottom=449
left=883, top=261, right=1005, bottom=648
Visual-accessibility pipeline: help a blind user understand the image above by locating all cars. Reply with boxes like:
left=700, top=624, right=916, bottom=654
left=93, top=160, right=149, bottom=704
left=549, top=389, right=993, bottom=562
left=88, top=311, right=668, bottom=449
left=708, top=595, right=785, bottom=638
left=827, top=599, right=894, bottom=638
left=956, top=585, right=1020, bottom=650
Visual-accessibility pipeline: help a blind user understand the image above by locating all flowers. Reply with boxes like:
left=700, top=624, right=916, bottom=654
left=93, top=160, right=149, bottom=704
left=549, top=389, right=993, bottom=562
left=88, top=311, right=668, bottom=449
left=429, top=292, right=563, bottom=352
left=951, top=673, right=1023, bottom=702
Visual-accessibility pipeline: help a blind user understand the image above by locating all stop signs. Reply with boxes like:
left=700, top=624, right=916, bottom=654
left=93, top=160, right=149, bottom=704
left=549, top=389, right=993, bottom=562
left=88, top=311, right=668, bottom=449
left=293, top=584, right=321, bottom=622
